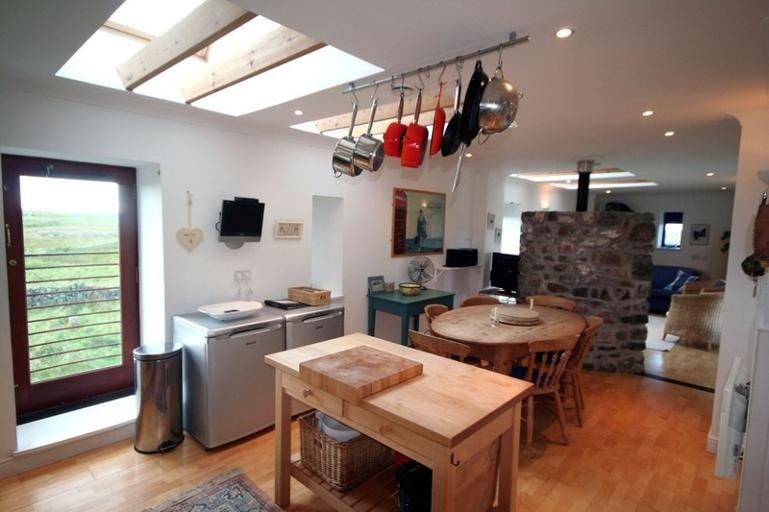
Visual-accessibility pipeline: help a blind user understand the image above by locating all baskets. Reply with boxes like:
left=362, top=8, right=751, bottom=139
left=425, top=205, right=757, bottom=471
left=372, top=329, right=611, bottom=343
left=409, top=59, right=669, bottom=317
left=297, top=411, right=396, bottom=492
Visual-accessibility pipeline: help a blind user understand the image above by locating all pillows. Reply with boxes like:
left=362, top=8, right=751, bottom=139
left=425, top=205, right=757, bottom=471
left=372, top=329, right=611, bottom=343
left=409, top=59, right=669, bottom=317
left=678, top=276, right=699, bottom=292
left=664, top=270, right=686, bottom=290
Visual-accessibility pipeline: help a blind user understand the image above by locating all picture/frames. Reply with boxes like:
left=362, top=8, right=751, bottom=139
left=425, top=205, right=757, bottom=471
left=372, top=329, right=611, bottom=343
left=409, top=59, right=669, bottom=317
left=391, top=187, right=445, bottom=257
left=368, top=275, right=384, bottom=293
left=690, top=224, right=710, bottom=245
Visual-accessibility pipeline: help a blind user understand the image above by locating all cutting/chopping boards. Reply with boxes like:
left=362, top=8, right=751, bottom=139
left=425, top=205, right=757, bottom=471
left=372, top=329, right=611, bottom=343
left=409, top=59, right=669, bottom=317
left=298, top=343, right=423, bottom=406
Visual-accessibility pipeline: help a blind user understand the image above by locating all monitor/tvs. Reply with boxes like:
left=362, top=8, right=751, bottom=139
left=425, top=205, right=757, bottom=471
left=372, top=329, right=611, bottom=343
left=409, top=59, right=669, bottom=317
left=490, top=252, right=520, bottom=295
left=219, top=197, right=265, bottom=242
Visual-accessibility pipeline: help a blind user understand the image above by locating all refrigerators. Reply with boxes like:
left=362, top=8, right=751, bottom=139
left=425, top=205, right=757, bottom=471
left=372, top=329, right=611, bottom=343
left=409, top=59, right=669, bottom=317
left=173, top=298, right=344, bottom=452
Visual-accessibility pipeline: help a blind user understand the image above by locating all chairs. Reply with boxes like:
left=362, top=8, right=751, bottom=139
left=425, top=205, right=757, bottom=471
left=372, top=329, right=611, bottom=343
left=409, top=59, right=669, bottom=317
left=461, top=297, right=499, bottom=306
left=424, top=304, right=448, bottom=335
left=527, top=296, right=575, bottom=311
left=663, top=279, right=727, bottom=350
left=512, top=334, right=580, bottom=459
left=408, top=329, right=471, bottom=362
left=536, top=315, right=604, bottom=428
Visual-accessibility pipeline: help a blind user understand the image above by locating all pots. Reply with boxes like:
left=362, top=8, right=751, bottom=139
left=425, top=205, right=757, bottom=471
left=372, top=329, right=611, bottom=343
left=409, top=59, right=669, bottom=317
left=331, top=59, right=519, bottom=180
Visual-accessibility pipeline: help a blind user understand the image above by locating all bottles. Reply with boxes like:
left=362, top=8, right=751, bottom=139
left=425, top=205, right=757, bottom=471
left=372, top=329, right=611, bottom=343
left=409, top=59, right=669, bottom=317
left=490, top=307, right=500, bottom=327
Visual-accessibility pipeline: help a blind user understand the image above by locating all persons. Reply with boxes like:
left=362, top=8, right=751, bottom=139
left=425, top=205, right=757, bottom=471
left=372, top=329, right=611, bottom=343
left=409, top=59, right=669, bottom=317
left=416, top=210, right=428, bottom=247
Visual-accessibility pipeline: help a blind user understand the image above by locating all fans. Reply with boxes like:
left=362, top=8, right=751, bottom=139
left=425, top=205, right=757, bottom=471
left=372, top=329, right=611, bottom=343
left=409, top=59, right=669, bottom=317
left=408, top=256, right=434, bottom=289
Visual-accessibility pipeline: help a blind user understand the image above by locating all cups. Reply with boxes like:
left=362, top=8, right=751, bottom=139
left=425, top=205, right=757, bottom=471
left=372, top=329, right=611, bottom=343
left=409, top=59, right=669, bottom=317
left=384, top=282, right=395, bottom=294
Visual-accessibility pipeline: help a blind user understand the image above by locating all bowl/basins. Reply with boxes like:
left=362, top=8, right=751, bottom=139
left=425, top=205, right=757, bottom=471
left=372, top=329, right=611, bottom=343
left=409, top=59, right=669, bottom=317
left=197, top=299, right=264, bottom=320
left=398, top=282, right=421, bottom=297
left=315, top=411, right=363, bottom=442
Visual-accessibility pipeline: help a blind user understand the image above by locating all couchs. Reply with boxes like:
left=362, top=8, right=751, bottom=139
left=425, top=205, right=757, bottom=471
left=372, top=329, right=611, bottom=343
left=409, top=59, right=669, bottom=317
left=649, top=265, right=699, bottom=316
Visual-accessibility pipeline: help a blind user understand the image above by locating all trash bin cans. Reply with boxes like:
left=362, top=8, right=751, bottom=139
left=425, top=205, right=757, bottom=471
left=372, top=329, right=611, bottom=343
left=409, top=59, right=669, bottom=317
left=132, top=341, right=185, bottom=454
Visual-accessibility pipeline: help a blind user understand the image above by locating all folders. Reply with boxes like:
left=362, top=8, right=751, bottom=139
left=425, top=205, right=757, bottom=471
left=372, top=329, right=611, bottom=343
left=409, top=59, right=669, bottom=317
left=264, top=299, right=311, bottom=310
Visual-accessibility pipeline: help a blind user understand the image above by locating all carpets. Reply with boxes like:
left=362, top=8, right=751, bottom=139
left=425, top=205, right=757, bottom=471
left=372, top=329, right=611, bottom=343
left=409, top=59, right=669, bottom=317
left=645, top=313, right=680, bottom=352
left=142, top=466, right=284, bottom=512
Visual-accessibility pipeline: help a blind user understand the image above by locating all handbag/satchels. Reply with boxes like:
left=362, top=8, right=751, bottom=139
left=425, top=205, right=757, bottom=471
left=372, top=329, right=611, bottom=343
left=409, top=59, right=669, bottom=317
left=395, top=460, right=432, bottom=512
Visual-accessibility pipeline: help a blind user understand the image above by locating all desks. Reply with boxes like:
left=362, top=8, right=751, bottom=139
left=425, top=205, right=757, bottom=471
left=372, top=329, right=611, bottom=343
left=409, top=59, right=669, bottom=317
left=431, top=304, right=586, bottom=374
left=366, top=287, right=456, bottom=346
left=263, top=332, right=535, bottom=512
